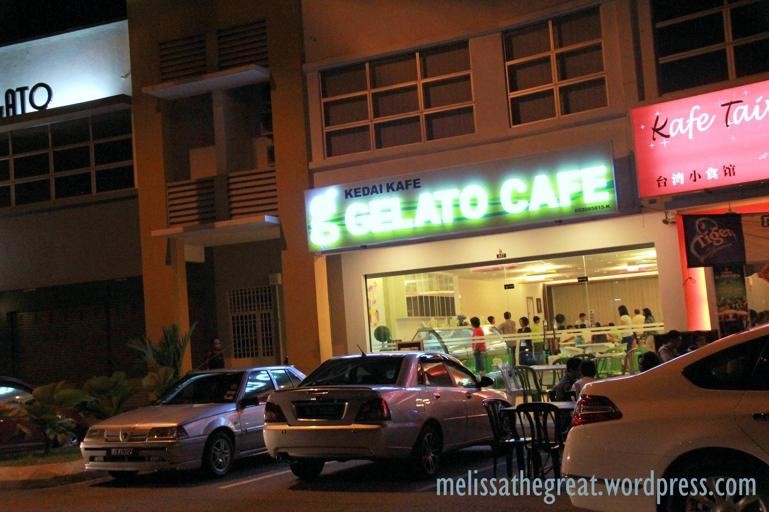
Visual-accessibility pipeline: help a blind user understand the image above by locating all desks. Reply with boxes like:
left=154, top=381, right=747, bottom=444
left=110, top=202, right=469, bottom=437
left=500, top=402, right=580, bottom=474
left=516, top=365, right=567, bottom=415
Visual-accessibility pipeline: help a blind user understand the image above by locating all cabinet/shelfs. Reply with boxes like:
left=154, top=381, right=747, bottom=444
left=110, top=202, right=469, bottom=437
left=410, top=324, right=512, bottom=380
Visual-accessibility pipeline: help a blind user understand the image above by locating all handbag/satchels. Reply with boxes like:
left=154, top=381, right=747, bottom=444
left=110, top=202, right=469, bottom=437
left=519, top=350, right=533, bottom=365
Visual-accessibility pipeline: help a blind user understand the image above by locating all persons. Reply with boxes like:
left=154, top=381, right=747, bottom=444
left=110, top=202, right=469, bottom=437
left=486, top=305, right=654, bottom=365
left=205, top=337, right=225, bottom=372
left=550, top=330, right=707, bottom=442
left=470, top=317, right=486, bottom=377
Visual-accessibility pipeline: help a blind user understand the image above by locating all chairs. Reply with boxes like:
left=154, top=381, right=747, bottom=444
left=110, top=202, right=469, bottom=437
left=515, top=365, right=551, bottom=419
left=549, top=333, right=660, bottom=376
left=482, top=398, right=535, bottom=479
left=547, top=357, right=570, bottom=402
left=498, top=364, right=533, bottom=406
left=516, top=403, right=561, bottom=486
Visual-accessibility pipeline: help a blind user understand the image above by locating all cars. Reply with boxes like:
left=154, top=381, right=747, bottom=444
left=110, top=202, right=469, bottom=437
left=562, top=322, right=768, bottom=512
left=260, top=347, right=516, bottom=483
left=79, top=365, right=308, bottom=480
left=0, top=378, right=86, bottom=456
